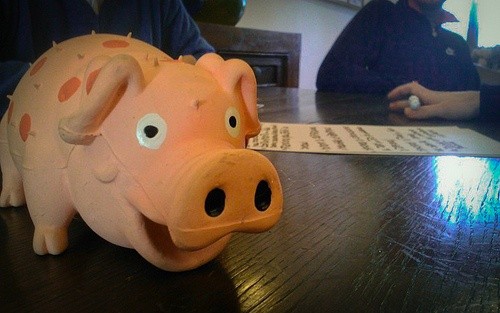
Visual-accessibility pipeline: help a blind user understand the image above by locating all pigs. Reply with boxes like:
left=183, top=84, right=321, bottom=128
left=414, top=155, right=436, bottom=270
left=0, top=30, right=284, bottom=271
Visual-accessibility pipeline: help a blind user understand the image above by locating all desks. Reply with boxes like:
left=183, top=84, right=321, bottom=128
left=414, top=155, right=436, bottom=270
left=0, top=87, right=500, bottom=313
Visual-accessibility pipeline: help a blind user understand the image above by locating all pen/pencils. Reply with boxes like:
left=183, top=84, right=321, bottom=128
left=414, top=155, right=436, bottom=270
left=408, top=96, right=420, bottom=109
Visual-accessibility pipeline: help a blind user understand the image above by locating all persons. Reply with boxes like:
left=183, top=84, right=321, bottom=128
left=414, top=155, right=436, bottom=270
left=1, top=1, right=215, bottom=98
left=389, top=82, right=500, bottom=125
left=316, top=0, right=481, bottom=97
